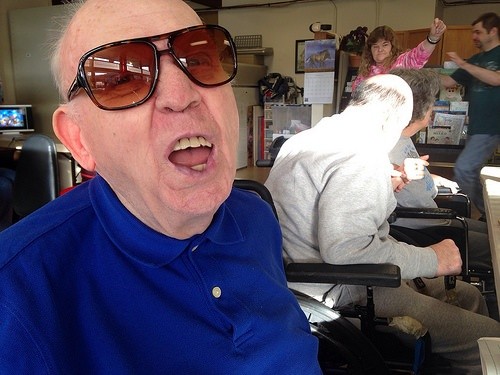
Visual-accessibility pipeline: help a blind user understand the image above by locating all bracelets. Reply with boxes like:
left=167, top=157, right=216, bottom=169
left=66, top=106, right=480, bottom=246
left=427, top=37, right=437, bottom=43
left=426, top=35, right=441, bottom=44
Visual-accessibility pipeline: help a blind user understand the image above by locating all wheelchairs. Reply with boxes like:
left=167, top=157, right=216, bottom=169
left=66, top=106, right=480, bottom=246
left=233, top=158, right=495, bottom=375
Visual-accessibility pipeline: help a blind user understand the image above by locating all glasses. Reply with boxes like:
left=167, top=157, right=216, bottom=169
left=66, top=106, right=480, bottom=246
left=66, top=24, right=238, bottom=111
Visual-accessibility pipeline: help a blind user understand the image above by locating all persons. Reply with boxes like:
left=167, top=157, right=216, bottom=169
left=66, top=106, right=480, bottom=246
left=261, top=67, right=500, bottom=375
left=454, top=12, right=500, bottom=222
left=0, top=1, right=326, bottom=375
left=351, top=18, right=447, bottom=91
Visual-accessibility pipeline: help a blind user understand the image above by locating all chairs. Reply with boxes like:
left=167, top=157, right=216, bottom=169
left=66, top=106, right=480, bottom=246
left=0, top=132, right=470, bottom=375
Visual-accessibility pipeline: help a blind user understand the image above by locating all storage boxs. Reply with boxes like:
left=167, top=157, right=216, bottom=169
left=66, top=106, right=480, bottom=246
left=271, top=106, right=312, bottom=134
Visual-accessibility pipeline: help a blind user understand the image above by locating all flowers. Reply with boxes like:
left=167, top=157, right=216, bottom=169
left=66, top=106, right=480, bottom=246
left=338, top=25, right=369, bottom=56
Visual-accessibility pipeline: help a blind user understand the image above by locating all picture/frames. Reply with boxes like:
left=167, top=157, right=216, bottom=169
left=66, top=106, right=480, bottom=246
left=295, top=38, right=315, bottom=74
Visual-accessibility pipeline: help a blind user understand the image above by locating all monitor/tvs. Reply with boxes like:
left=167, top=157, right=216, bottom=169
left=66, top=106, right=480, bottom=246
left=0, top=105, right=35, bottom=135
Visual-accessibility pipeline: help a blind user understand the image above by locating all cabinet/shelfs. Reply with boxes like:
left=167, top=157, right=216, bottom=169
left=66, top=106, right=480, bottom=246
left=264, top=102, right=301, bottom=163
